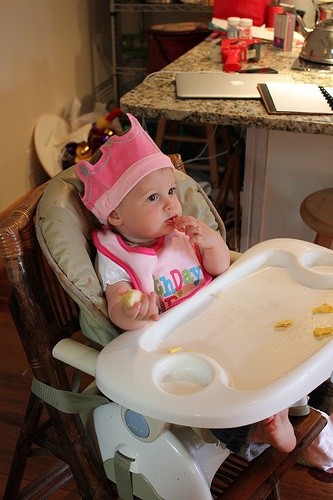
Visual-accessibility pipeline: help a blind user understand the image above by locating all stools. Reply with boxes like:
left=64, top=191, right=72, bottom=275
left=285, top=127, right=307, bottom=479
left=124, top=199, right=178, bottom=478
left=299, top=188, right=333, bottom=250
left=155, top=117, right=232, bottom=189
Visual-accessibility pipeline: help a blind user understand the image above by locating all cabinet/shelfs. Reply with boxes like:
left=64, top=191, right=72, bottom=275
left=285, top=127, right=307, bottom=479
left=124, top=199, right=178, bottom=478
left=108, top=0, right=213, bottom=175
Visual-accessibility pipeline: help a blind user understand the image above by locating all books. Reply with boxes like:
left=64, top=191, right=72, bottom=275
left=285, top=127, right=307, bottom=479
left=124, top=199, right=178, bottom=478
left=255, top=82, right=333, bottom=114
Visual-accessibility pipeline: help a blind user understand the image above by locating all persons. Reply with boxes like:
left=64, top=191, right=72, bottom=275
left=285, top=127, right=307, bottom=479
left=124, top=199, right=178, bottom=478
left=83, top=138, right=310, bottom=453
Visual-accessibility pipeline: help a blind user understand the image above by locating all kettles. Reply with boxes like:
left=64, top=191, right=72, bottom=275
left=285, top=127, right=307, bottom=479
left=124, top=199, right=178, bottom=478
left=296, top=15, right=333, bottom=65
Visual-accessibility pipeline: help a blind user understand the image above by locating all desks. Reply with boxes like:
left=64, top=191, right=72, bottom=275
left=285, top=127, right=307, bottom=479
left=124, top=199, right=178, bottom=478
left=120, top=29, right=333, bottom=254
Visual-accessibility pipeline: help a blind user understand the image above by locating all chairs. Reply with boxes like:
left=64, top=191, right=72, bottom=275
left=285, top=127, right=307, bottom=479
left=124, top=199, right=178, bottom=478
left=0, top=153, right=333, bottom=500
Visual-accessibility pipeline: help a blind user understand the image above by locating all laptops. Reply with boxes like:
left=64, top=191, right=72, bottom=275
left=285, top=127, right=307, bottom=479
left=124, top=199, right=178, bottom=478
left=176, top=72, right=295, bottom=98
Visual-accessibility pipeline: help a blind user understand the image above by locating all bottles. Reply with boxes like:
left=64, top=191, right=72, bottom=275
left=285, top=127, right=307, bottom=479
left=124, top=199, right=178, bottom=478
left=240, top=18, right=253, bottom=39
left=228, top=17, right=240, bottom=39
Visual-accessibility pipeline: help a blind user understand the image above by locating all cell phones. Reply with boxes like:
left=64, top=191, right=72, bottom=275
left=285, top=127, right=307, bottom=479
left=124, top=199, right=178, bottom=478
left=235, top=67, right=278, bottom=73
